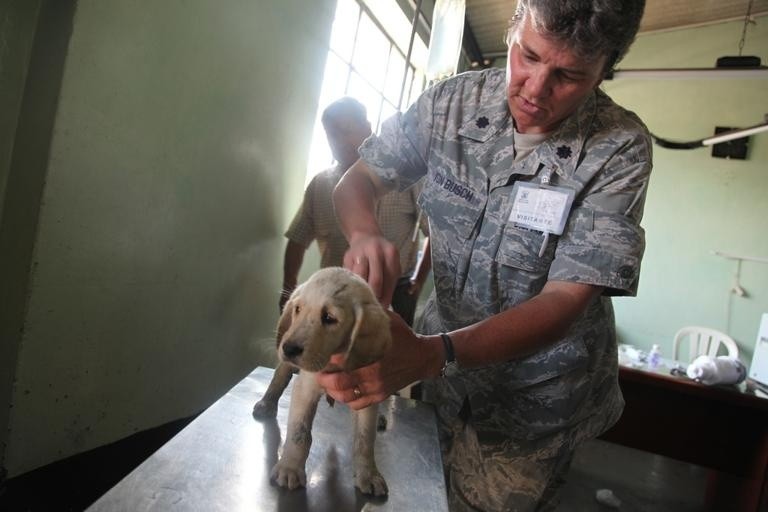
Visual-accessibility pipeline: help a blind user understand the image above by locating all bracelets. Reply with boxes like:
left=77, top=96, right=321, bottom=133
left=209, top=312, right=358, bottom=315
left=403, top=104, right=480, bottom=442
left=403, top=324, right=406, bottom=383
left=438, top=332, right=455, bottom=364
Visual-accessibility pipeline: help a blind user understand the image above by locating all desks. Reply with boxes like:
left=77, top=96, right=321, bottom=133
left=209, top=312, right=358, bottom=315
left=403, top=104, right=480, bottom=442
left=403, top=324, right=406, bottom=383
left=599, top=346, right=767, bottom=512
left=87, top=366, right=448, bottom=512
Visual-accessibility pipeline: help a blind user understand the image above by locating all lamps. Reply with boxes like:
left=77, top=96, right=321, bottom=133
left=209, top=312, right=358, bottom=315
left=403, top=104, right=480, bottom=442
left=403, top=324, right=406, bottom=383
left=608, top=0, right=767, bottom=81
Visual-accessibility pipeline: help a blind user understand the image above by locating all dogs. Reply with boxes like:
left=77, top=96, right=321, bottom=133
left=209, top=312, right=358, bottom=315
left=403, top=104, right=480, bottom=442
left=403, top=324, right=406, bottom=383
left=252, top=267, right=394, bottom=497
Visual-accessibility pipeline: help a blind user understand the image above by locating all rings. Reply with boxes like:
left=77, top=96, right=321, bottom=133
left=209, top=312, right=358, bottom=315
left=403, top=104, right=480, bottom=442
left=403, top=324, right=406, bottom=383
left=351, top=383, right=364, bottom=399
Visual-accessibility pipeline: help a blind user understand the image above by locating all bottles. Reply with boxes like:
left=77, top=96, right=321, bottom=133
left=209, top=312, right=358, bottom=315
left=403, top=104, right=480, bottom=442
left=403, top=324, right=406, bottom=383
left=648, top=344, right=662, bottom=369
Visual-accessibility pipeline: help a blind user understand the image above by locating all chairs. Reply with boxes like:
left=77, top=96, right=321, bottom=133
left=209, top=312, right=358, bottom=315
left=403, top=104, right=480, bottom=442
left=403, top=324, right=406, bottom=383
left=673, top=325, right=739, bottom=373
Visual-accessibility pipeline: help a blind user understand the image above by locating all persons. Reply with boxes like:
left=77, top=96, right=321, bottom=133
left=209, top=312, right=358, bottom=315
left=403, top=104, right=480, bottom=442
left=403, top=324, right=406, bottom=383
left=273, top=96, right=434, bottom=402
left=327, top=0, right=657, bottom=512
